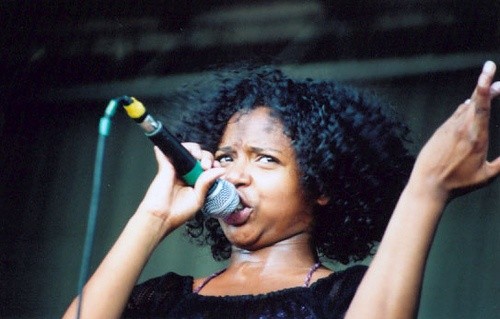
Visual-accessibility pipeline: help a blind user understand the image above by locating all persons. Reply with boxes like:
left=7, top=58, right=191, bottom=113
left=61, top=60, right=500, bottom=319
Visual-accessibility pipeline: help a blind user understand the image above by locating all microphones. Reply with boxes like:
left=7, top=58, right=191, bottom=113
left=123, top=96, right=240, bottom=218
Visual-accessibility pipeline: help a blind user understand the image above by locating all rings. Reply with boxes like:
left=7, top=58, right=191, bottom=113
left=464, top=98, right=472, bottom=104
